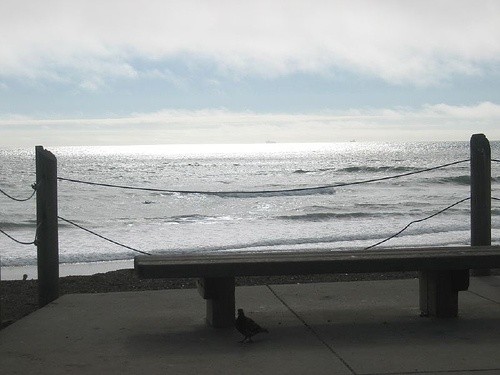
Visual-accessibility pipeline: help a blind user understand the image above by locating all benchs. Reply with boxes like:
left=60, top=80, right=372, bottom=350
left=134, top=246, right=500, bottom=326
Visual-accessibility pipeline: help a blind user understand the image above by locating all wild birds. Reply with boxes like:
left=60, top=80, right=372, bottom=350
left=234, top=309, right=269, bottom=343
